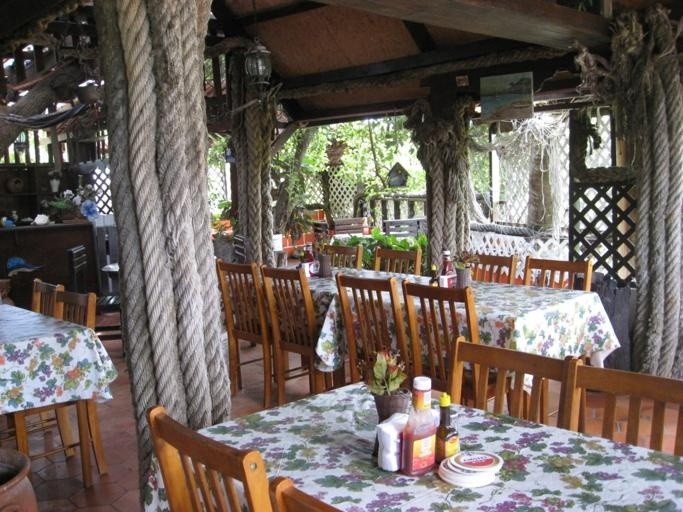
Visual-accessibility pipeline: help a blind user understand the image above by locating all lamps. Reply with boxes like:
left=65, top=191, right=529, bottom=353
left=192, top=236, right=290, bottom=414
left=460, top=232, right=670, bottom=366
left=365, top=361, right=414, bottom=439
left=239, top=0, right=275, bottom=94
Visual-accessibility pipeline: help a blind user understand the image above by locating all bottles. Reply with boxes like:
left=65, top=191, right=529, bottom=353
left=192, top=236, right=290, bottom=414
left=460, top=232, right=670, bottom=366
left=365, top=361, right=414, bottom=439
left=399, top=375, right=436, bottom=476
left=3, top=210, right=33, bottom=229
left=210, top=218, right=233, bottom=241
left=294, top=245, right=318, bottom=277
left=428, top=249, right=472, bottom=290
left=434, top=391, right=459, bottom=464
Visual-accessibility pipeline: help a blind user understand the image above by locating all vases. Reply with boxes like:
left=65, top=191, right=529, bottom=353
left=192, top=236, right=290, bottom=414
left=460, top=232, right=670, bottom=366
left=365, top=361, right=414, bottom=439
left=49, top=178, right=60, bottom=193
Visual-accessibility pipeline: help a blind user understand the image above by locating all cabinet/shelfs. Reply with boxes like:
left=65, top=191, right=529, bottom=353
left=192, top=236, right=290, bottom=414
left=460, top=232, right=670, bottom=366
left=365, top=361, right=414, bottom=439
left=0, top=164, right=78, bottom=225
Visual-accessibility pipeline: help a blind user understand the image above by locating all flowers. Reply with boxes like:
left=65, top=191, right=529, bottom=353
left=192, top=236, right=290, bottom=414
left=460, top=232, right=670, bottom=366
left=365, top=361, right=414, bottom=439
left=48, top=169, right=63, bottom=180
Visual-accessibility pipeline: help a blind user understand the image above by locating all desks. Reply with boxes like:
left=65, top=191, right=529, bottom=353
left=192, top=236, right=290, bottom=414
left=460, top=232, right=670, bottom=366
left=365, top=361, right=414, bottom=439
left=0, top=220, right=102, bottom=316
